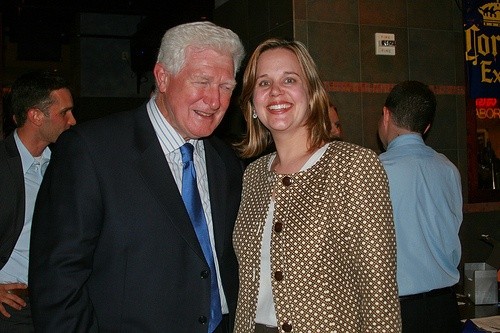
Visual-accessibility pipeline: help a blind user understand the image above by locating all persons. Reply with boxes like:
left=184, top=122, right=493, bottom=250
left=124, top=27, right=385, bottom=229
left=0, top=68, right=76, bottom=332
left=378, top=80, right=463, bottom=333
left=27, top=21, right=245, bottom=333
left=232, top=38, right=402, bottom=333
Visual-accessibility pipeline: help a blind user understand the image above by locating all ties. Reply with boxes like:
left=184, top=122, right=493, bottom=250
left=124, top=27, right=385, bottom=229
left=179, top=143, right=222, bottom=333
